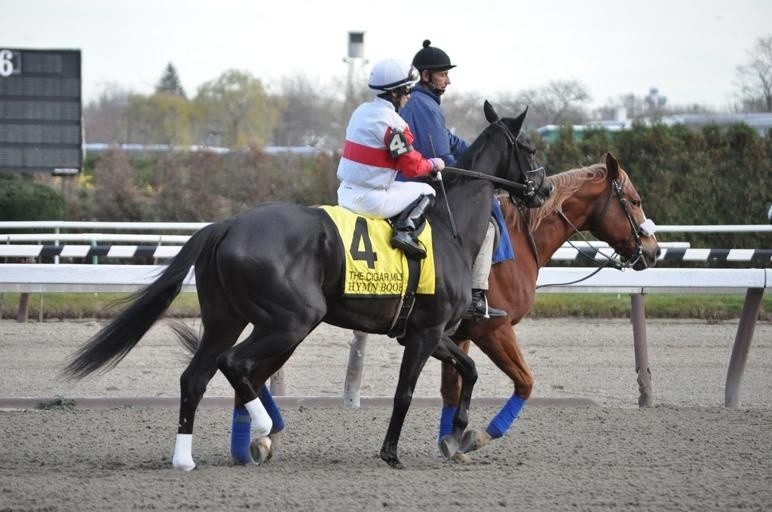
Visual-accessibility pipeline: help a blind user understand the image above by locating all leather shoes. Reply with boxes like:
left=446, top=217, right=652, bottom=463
left=467, top=298, right=507, bottom=317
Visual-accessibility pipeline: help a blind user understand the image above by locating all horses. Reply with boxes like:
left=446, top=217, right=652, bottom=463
left=166, top=152, right=661, bottom=465
left=59, top=99, right=554, bottom=472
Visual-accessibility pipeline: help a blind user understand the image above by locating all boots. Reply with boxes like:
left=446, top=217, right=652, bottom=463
left=389, top=194, right=438, bottom=259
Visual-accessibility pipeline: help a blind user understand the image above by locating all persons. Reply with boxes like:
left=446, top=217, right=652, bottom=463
left=336, top=59, right=446, bottom=261
left=391, top=41, right=518, bottom=322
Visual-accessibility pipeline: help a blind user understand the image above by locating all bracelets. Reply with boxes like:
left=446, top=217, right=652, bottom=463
left=429, top=157, right=437, bottom=173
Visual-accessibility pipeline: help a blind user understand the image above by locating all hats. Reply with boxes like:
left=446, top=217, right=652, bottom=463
left=412, top=39, right=457, bottom=71
left=367, top=57, right=420, bottom=94
